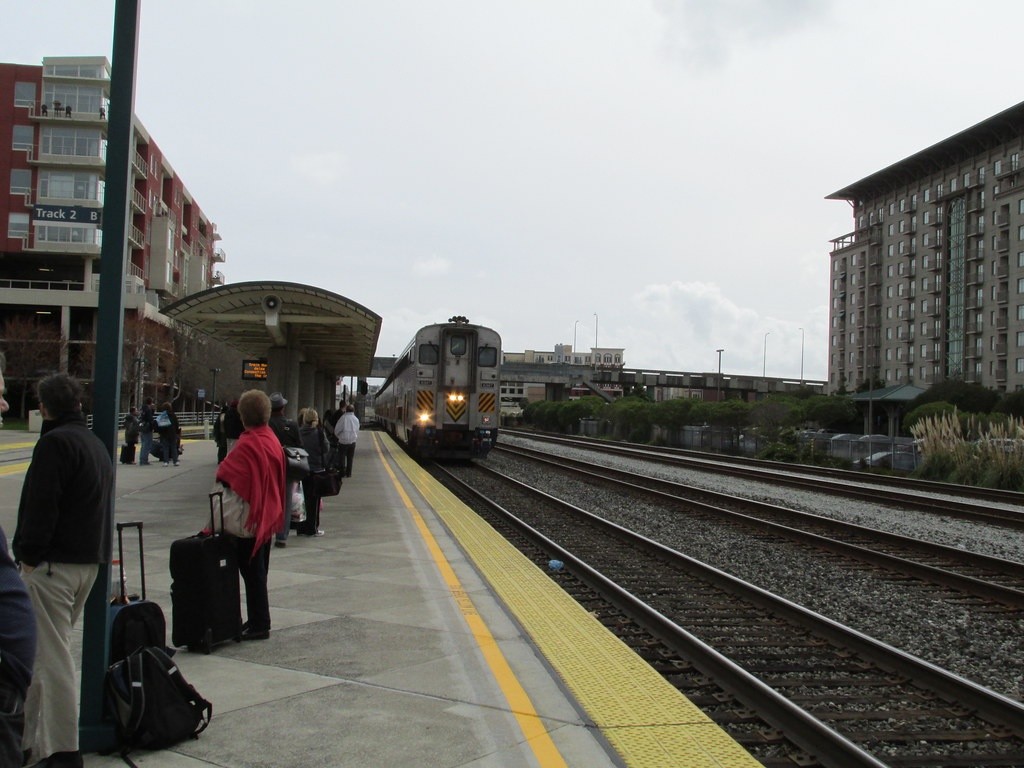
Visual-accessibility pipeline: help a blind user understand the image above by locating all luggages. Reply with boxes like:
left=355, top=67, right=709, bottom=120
left=169, top=493, right=244, bottom=654
left=111, top=521, right=166, bottom=663
left=120, top=439, right=135, bottom=463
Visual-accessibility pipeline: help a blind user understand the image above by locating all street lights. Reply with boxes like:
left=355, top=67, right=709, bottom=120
left=211, top=368, right=221, bottom=424
left=764, top=332, right=770, bottom=377
left=135, top=357, right=147, bottom=411
left=574, top=320, right=579, bottom=355
left=798, top=327, right=804, bottom=383
left=593, top=313, right=597, bottom=348
left=717, top=349, right=726, bottom=402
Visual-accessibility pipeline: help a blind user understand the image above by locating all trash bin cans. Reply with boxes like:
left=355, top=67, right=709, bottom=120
left=29, top=410, right=43, bottom=433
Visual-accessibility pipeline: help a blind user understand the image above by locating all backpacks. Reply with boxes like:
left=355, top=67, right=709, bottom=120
left=106, top=646, right=213, bottom=752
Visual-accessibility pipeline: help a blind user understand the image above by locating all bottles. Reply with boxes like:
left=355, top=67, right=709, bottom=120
left=112, top=559, right=128, bottom=599
left=547, top=560, right=563, bottom=569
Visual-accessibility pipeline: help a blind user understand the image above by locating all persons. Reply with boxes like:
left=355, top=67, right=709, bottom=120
left=324, top=400, right=347, bottom=464
left=0, top=352, right=37, bottom=768
left=297, top=406, right=331, bottom=537
left=334, top=404, right=359, bottom=478
left=139, top=396, right=155, bottom=465
left=124, top=407, right=139, bottom=464
left=213, top=401, right=244, bottom=465
left=268, top=391, right=303, bottom=548
left=157, top=402, right=181, bottom=467
left=12, top=373, right=114, bottom=768
left=204, top=389, right=286, bottom=640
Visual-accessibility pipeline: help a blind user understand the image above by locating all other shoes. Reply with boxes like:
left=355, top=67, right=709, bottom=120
left=232, top=627, right=270, bottom=639
left=242, top=621, right=271, bottom=630
left=174, top=462, right=179, bottom=465
left=306, top=531, right=324, bottom=537
left=297, top=531, right=306, bottom=535
left=275, top=542, right=286, bottom=547
left=22, top=757, right=84, bottom=768
left=162, top=463, right=167, bottom=466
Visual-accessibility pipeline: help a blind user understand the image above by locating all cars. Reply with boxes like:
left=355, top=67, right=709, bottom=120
left=849, top=435, right=897, bottom=451
left=855, top=451, right=920, bottom=472
left=990, top=438, right=1016, bottom=454
left=831, top=434, right=860, bottom=446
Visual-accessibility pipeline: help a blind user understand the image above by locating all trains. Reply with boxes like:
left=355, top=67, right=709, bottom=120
left=374, top=315, right=503, bottom=460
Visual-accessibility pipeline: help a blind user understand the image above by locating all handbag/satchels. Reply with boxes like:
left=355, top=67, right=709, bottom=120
left=156, top=410, right=171, bottom=426
left=149, top=438, right=161, bottom=457
left=313, top=466, right=343, bottom=495
left=291, top=481, right=306, bottom=522
left=137, top=421, right=150, bottom=433
left=282, top=445, right=309, bottom=475
left=203, top=480, right=257, bottom=538
left=324, top=416, right=334, bottom=434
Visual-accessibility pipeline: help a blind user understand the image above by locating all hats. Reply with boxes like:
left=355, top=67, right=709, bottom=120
left=230, top=400, right=238, bottom=407
left=270, top=392, right=288, bottom=408
